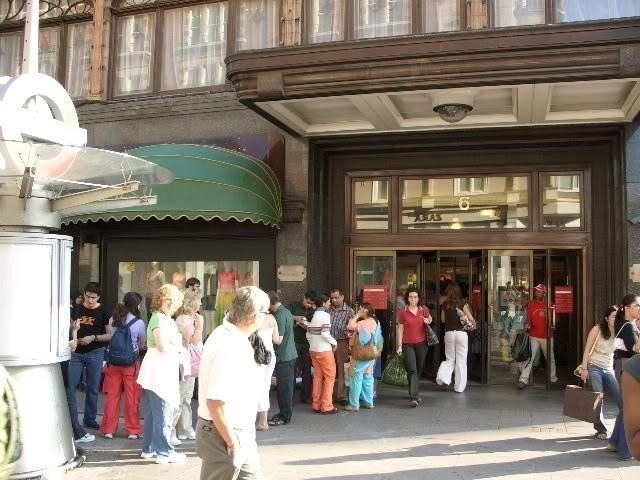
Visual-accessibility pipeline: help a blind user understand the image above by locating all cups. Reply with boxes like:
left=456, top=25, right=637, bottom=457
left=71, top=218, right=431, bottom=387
left=628, top=304, right=639, bottom=309
left=259, top=310, right=269, bottom=316
left=306, top=299, right=315, bottom=305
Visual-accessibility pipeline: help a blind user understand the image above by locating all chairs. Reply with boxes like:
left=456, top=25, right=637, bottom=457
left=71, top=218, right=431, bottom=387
left=345, top=405, right=359, bottom=411
left=341, top=400, right=347, bottom=406
left=127, top=435, right=137, bottom=439
left=598, top=434, right=607, bottom=440
left=75, top=433, right=95, bottom=443
left=178, top=431, right=196, bottom=439
left=171, top=437, right=182, bottom=446
left=373, top=390, right=378, bottom=399
left=84, top=421, right=100, bottom=430
left=359, top=401, right=375, bottom=408
left=517, top=381, right=526, bottom=390
left=156, top=450, right=186, bottom=464
left=305, top=399, right=312, bottom=404
left=410, top=400, right=418, bottom=407
left=105, top=433, right=114, bottom=439
left=141, top=451, right=157, bottom=458
left=256, top=423, right=269, bottom=432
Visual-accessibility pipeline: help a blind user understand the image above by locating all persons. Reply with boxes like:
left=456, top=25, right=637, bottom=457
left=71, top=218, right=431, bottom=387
left=172, top=262, right=191, bottom=290
left=255, top=313, right=279, bottom=431
left=620, top=354, right=640, bottom=460
left=137, top=284, right=184, bottom=464
left=169, top=288, right=203, bottom=445
left=343, top=302, right=380, bottom=411
left=62, top=282, right=112, bottom=443
left=174, top=279, right=203, bottom=319
left=604, top=293, right=640, bottom=460
left=306, top=295, right=338, bottom=415
left=215, top=260, right=240, bottom=328
left=266, top=291, right=298, bottom=426
left=145, top=261, right=166, bottom=323
left=98, top=292, right=146, bottom=439
left=283, top=290, right=318, bottom=407
left=240, top=272, right=253, bottom=287
left=382, top=278, right=562, bottom=408
left=195, top=285, right=270, bottom=478
left=574, top=305, right=624, bottom=439
left=200, top=261, right=220, bottom=338
left=325, top=288, right=357, bottom=406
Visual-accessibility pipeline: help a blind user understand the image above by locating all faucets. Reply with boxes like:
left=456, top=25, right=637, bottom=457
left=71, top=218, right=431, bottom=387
left=323, top=408, right=339, bottom=415
left=269, top=420, right=285, bottom=426
left=272, top=413, right=281, bottom=419
left=312, top=409, right=321, bottom=412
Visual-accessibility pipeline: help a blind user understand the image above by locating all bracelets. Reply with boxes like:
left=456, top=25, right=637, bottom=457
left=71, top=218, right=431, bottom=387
left=94, top=334, right=98, bottom=342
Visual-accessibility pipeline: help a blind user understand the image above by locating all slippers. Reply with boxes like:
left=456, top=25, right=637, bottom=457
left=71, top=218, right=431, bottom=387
left=108, top=316, right=141, bottom=366
left=511, top=329, right=532, bottom=368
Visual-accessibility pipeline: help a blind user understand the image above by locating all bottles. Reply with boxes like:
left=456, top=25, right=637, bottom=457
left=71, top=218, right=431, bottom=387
left=533, top=284, right=546, bottom=294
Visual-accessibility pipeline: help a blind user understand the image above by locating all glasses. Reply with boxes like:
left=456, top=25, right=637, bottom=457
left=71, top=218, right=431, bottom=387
left=433, top=103, right=474, bottom=124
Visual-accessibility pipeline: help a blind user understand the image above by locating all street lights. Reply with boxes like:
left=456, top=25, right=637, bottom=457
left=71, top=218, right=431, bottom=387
left=574, top=361, right=582, bottom=377
left=351, top=334, right=377, bottom=360
left=613, top=337, right=636, bottom=351
left=563, top=384, right=604, bottom=423
left=191, top=345, right=201, bottom=376
left=461, top=303, right=475, bottom=331
left=381, top=352, right=409, bottom=386
left=426, top=323, right=439, bottom=346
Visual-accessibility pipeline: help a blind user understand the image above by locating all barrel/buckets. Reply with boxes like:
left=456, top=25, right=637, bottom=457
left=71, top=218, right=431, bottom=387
left=606, top=444, right=618, bottom=452
